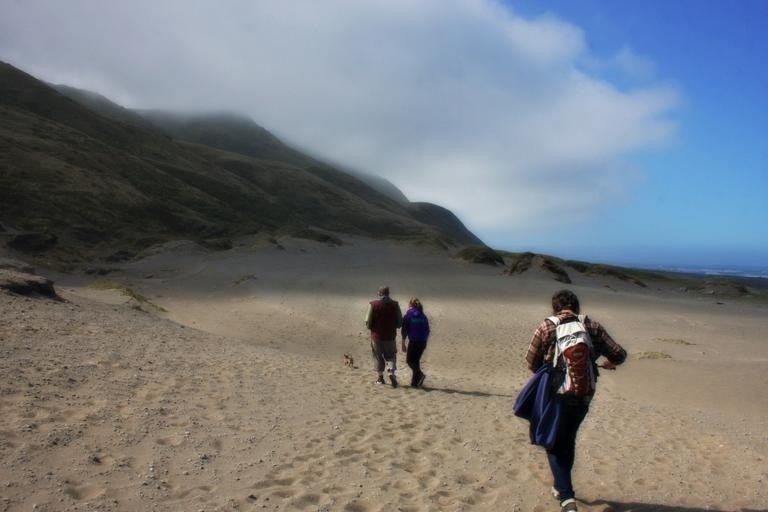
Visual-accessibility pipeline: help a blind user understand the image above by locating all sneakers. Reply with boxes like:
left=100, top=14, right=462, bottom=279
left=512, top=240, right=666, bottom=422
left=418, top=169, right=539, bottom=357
left=552, top=485, right=576, bottom=512
left=376, top=372, right=425, bottom=387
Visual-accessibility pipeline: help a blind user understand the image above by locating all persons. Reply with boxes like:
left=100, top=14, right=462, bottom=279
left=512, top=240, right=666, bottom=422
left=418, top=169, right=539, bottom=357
left=526, top=289, right=627, bottom=512
left=365, top=286, right=401, bottom=388
left=401, top=297, right=429, bottom=387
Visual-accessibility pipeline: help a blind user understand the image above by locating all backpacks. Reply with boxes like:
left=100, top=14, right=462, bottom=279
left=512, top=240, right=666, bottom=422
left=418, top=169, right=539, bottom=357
left=545, top=314, right=596, bottom=396
